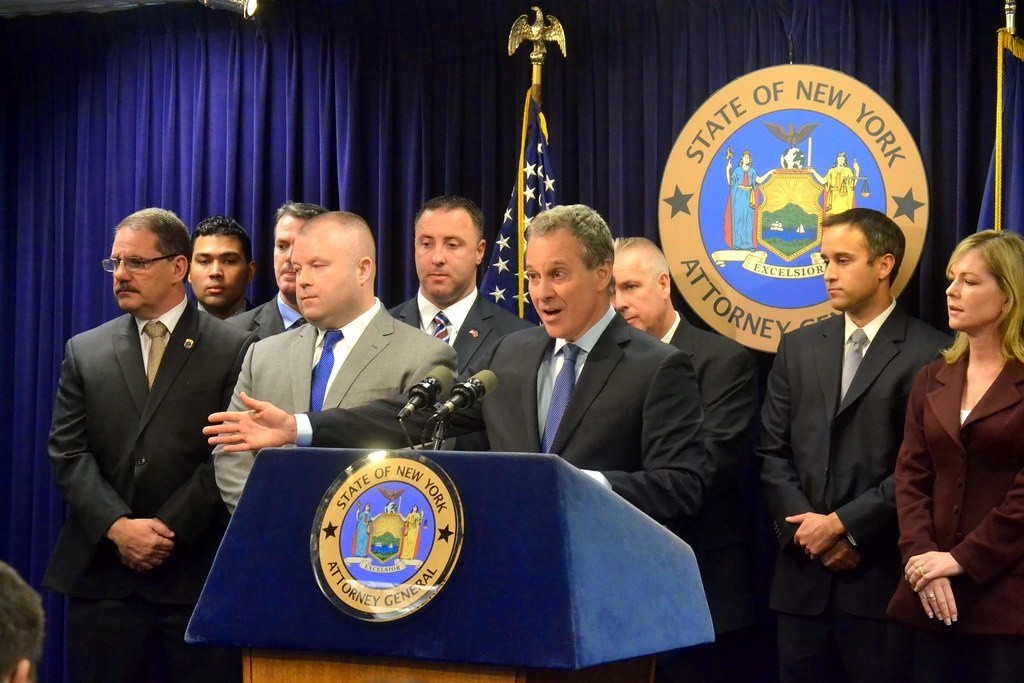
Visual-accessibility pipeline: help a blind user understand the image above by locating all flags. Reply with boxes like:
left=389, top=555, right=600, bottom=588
left=478, top=85, right=563, bottom=324
left=975, top=26, right=1024, bottom=239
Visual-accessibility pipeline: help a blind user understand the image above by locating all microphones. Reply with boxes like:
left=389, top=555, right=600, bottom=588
left=397, top=365, right=453, bottom=421
left=434, top=370, right=498, bottom=419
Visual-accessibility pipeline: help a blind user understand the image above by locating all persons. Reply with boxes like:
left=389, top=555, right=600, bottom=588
left=385, top=194, right=540, bottom=384
left=39, top=207, right=260, bottom=683
left=884, top=227, right=1024, bottom=683
left=0, top=559, right=47, bottom=683
left=609, top=236, right=756, bottom=683
left=202, top=204, right=711, bottom=530
left=213, top=210, right=460, bottom=515
left=755, top=207, right=955, bottom=683
left=186, top=198, right=332, bottom=340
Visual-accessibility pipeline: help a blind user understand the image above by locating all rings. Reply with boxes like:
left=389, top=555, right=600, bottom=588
left=918, top=566, right=926, bottom=577
left=926, top=592, right=935, bottom=599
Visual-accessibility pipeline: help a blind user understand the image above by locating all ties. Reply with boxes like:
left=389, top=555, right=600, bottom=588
left=542, top=342, right=580, bottom=456
left=309, top=331, right=344, bottom=413
left=433, top=311, right=450, bottom=344
left=143, top=321, right=168, bottom=393
left=841, top=329, right=869, bottom=403
left=297, top=318, right=306, bottom=326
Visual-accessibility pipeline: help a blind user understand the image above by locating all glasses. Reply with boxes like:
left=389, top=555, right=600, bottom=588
left=101, top=252, right=183, bottom=272
